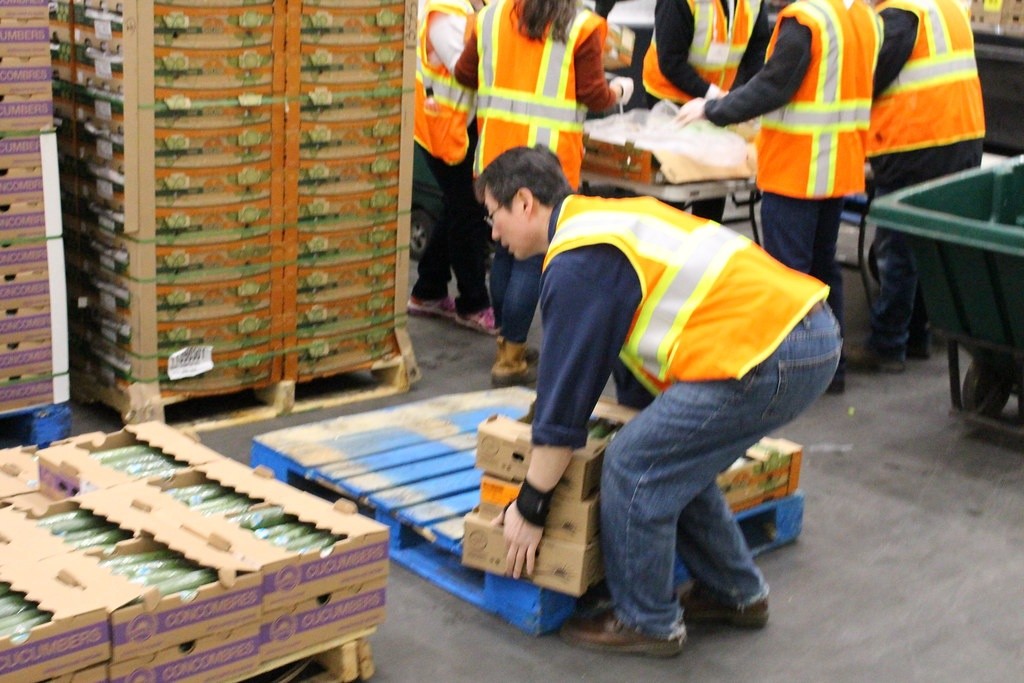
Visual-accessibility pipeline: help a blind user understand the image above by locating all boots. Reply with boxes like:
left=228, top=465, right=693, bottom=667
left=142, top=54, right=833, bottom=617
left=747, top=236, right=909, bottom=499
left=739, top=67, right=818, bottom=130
left=491, top=336, right=538, bottom=389
left=524, top=348, right=539, bottom=367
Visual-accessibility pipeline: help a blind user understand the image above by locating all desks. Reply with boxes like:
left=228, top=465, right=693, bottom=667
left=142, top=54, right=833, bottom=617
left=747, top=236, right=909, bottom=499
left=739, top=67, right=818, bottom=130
left=583, top=172, right=762, bottom=246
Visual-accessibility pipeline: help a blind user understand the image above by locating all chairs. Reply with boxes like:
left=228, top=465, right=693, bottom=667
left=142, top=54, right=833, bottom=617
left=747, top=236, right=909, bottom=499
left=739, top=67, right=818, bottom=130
left=834, top=178, right=876, bottom=313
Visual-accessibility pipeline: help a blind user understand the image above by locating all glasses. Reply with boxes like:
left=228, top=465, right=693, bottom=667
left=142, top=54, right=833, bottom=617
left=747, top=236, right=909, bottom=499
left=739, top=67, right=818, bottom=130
left=484, top=205, right=502, bottom=226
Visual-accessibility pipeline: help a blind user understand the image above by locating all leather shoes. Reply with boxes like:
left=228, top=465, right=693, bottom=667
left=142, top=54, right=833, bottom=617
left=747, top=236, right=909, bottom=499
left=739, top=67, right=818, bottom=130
left=560, top=607, right=684, bottom=657
left=680, top=589, right=770, bottom=628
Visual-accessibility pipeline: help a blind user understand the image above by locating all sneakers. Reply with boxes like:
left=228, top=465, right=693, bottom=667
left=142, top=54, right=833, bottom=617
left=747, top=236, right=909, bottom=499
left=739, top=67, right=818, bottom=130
left=406, top=295, right=456, bottom=318
left=455, top=306, right=496, bottom=335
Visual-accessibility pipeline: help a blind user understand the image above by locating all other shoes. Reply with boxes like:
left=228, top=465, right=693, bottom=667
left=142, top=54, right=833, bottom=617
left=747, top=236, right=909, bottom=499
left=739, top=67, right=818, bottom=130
left=844, top=344, right=905, bottom=373
left=905, top=344, right=932, bottom=360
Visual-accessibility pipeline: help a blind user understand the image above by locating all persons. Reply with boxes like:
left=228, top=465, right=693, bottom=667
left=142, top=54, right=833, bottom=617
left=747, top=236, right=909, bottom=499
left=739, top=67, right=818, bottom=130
left=674, top=0, right=885, bottom=392
left=473, top=144, right=846, bottom=657
left=580, top=0, right=657, bottom=115
left=841, top=0, right=986, bottom=374
left=642, top=0, right=771, bottom=223
left=405, top=0, right=502, bottom=336
left=454, top=0, right=634, bottom=387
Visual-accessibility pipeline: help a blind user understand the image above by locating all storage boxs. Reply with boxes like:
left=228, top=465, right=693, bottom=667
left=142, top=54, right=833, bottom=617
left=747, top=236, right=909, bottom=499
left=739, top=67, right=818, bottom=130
left=717, top=436, right=805, bottom=513
left=478, top=471, right=600, bottom=546
left=476, top=399, right=638, bottom=500
left=604, top=25, right=636, bottom=70
left=582, top=140, right=667, bottom=188
left=463, top=506, right=605, bottom=598
left=0, top=0, right=412, bottom=683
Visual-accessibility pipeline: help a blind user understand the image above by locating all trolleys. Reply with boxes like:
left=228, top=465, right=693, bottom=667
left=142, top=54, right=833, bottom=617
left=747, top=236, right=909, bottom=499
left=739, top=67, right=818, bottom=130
left=862, top=153, right=1024, bottom=439
